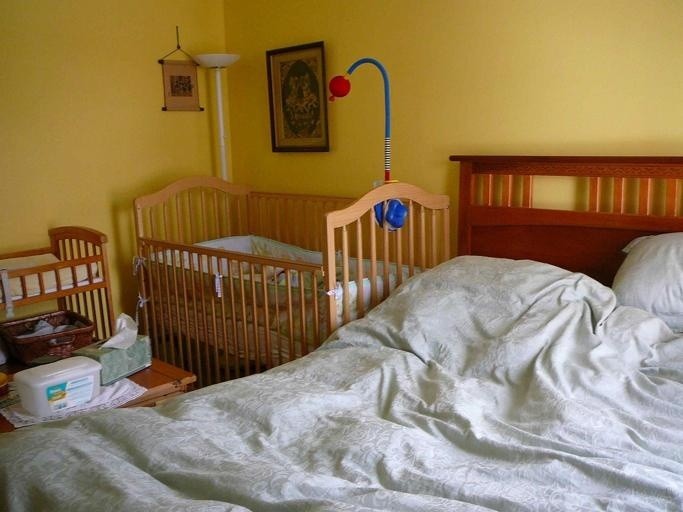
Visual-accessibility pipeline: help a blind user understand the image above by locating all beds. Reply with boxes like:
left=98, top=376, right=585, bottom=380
left=1, top=154, right=683, bottom=511
left=130, top=172, right=451, bottom=396
left=1, top=223, right=114, bottom=342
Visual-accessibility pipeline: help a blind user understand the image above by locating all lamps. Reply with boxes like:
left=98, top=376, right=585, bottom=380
left=195, top=52, right=242, bottom=181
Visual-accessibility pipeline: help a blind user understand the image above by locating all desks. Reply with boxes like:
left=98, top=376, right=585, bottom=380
left=0, top=338, right=197, bottom=434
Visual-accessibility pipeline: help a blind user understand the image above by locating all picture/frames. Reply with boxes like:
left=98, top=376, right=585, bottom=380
left=264, top=39, right=329, bottom=154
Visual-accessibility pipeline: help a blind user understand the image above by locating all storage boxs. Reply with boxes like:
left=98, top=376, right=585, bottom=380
left=11, top=354, right=103, bottom=419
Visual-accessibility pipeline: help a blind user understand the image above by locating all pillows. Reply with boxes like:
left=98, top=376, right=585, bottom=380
left=614, top=232, right=682, bottom=332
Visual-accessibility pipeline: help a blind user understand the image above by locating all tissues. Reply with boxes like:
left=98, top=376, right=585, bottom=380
left=70, top=312, right=153, bottom=387
